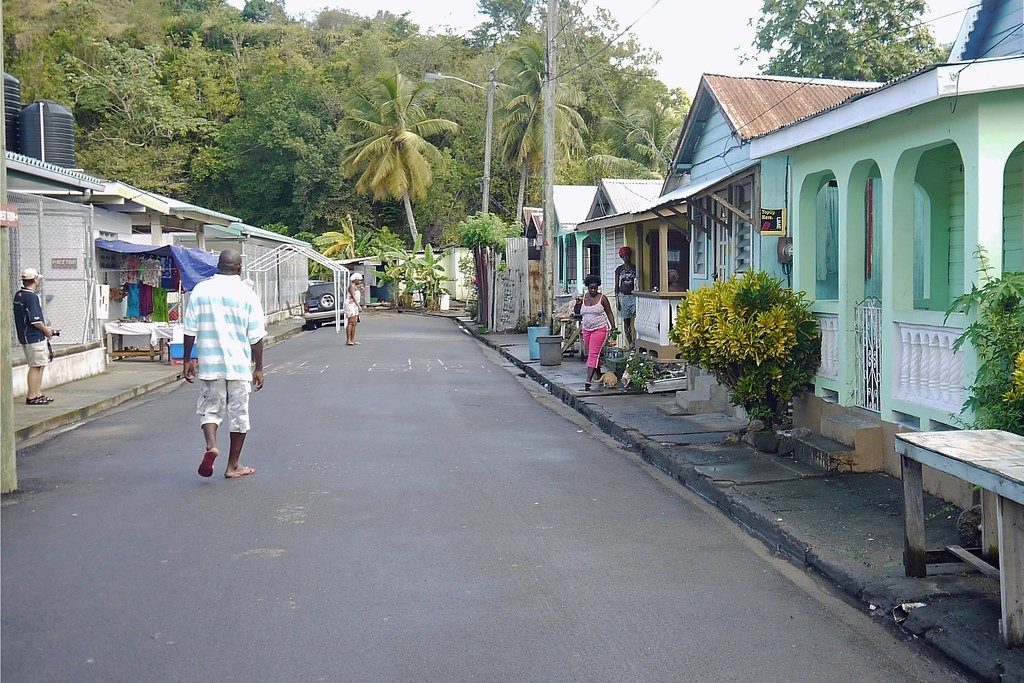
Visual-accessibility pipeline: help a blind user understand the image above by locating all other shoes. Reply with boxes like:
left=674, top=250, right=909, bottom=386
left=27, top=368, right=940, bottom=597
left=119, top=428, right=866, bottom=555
left=26, top=398, right=48, bottom=404
left=41, top=396, right=54, bottom=402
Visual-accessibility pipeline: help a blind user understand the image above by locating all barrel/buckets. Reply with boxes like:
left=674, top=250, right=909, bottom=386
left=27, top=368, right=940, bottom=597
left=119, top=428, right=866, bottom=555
left=527, top=327, right=549, bottom=359
left=536, top=336, right=563, bottom=365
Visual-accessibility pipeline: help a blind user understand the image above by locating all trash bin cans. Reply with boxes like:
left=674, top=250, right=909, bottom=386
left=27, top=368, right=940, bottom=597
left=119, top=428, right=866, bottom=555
left=537, top=335, right=564, bottom=366
left=527, top=326, right=550, bottom=360
left=438, top=292, right=450, bottom=311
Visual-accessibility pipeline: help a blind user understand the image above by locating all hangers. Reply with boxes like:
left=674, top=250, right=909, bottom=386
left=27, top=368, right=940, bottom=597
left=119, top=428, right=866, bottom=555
left=120, top=254, right=160, bottom=261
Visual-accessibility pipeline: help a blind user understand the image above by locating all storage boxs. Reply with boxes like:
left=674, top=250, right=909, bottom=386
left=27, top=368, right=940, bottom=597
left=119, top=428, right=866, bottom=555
left=169, top=341, right=197, bottom=358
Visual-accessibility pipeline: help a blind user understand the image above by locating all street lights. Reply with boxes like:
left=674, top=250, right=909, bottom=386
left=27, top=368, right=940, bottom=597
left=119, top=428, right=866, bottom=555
left=422, top=72, right=495, bottom=215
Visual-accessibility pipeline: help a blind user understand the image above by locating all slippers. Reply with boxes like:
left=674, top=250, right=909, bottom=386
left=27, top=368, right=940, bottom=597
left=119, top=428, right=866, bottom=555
left=233, top=467, right=254, bottom=477
left=198, top=451, right=215, bottom=477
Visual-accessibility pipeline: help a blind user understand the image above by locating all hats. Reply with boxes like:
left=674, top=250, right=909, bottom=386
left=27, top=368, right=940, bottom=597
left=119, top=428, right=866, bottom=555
left=22, top=268, right=43, bottom=280
left=350, top=273, right=363, bottom=281
left=619, top=246, right=632, bottom=257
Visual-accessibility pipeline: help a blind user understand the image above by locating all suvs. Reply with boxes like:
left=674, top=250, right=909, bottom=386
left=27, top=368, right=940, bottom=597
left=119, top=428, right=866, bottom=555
left=304, top=282, right=346, bottom=330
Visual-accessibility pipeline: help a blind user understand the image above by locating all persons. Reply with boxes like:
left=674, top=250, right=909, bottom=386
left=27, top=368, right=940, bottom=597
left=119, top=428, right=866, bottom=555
left=343, top=272, right=364, bottom=345
left=182, top=249, right=268, bottom=478
left=12, top=267, right=55, bottom=405
left=614, top=246, right=636, bottom=353
left=573, top=275, right=615, bottom=388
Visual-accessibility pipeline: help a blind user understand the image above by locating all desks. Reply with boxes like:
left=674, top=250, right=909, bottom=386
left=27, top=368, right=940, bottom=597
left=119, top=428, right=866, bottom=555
left=895, top=428, right=1024, bottom=649
left=561, top=314, right=604, bottom=361
left=105, top=320, right=173, bottom=362
